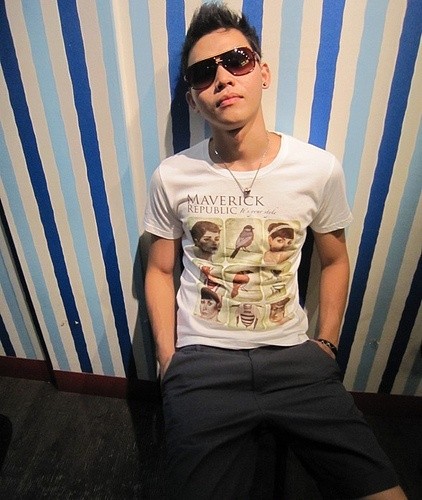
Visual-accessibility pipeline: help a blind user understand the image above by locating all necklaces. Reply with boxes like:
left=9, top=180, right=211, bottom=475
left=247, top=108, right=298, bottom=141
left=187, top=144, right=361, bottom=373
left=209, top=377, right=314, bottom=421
left=214, top=129, right=269, bottom=196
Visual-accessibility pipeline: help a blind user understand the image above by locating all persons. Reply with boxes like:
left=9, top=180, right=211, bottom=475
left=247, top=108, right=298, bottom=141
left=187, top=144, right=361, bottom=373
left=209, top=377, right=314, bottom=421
left=144, top=1, right=407, bottom=500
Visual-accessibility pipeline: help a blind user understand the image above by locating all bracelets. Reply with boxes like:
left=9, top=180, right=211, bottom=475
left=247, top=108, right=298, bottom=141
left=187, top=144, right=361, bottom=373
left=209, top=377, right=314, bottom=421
left=315, top=339, right=339, bottom=358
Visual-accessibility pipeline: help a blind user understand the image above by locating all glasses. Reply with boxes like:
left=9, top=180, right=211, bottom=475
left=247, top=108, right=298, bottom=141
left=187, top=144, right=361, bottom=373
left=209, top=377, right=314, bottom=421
left=184, top=48, right=262, bottom=90
